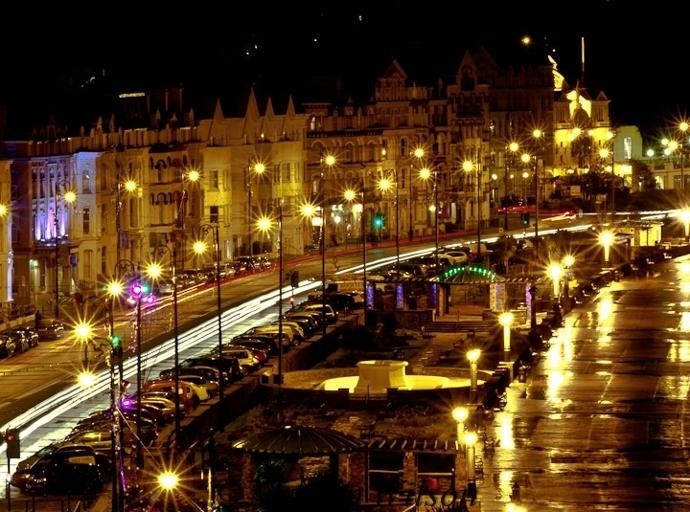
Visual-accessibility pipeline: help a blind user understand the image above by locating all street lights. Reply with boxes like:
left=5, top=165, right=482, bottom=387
left=246, top=144, right=365, bottom=371
